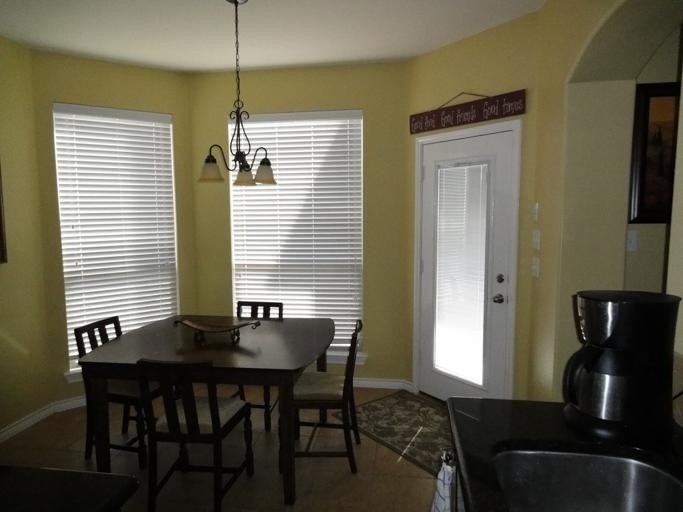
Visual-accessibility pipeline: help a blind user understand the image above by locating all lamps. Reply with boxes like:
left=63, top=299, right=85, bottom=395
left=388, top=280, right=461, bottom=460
left=198, top=1, right=277, bottom=186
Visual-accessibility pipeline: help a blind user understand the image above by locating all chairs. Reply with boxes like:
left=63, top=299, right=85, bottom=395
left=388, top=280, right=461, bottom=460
left=276, top=317, right=363, bottom=478
left=74, top=313, right=168, bottom=472
left=129, top=355, right=257, bottom=509
left=230, top=302, right=284, bottom=435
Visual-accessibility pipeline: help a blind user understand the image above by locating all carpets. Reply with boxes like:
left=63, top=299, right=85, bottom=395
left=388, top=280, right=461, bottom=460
left=332, top=388, right=453, bottom=477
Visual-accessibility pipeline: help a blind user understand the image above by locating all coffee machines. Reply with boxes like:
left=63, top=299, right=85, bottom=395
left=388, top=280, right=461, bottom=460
left=561, top=289, right=681, bottom=453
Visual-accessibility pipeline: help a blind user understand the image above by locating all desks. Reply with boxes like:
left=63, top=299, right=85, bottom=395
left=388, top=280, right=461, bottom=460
left=0, top=463, right=141, bottom=512
left=76, top=316, right=337, bottom=511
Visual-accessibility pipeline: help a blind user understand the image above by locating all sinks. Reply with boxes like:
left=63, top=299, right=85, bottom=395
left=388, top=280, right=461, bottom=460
left=471, top=437, right=683, bottom=512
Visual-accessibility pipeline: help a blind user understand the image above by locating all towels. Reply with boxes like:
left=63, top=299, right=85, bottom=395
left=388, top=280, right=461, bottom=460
left=430, top=463, right=456, bottom=512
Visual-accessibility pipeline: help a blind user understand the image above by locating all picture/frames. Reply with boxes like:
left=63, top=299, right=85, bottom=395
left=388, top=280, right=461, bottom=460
left=629, top=82, right=680, bottom=223
